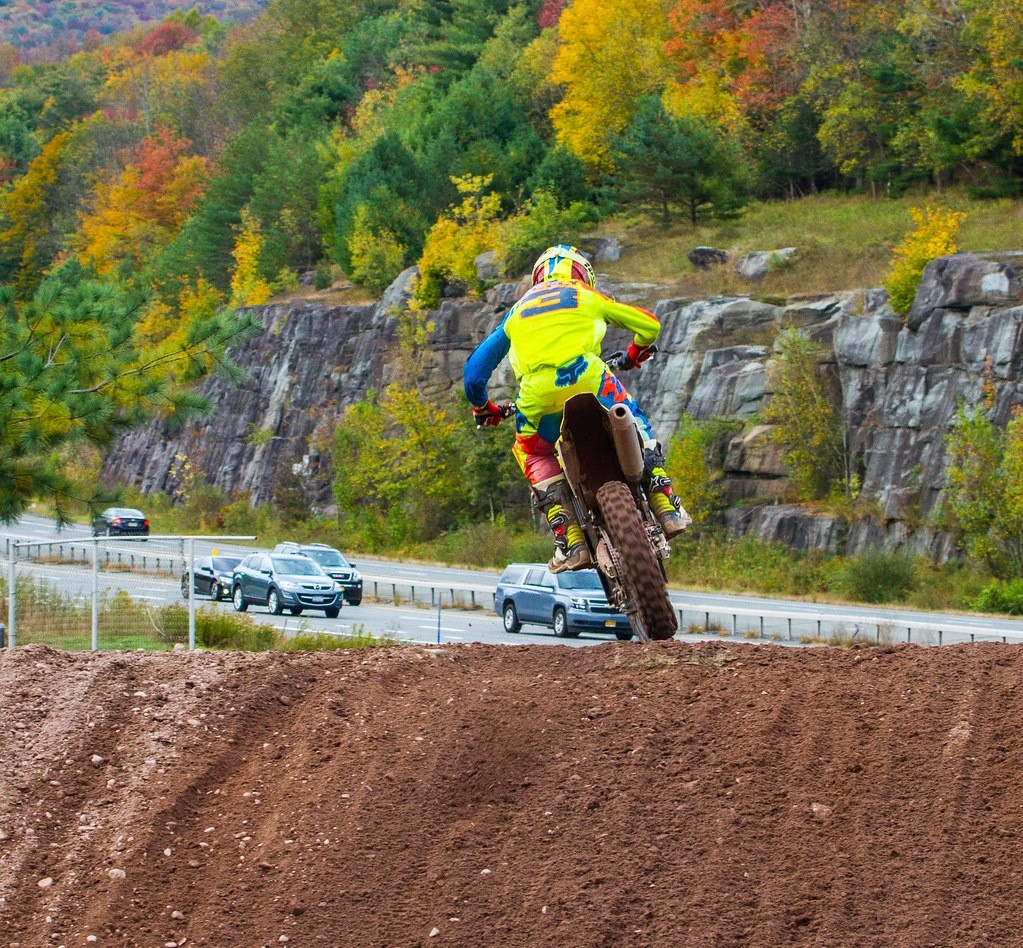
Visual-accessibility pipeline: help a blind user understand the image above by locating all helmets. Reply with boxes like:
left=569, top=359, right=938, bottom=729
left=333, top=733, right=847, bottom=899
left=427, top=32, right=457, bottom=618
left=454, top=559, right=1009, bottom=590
left=530, top=244, right=595, bottom=288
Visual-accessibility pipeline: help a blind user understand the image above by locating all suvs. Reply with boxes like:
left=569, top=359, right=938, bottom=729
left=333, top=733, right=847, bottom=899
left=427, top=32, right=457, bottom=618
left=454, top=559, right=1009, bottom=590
left=272, top=541, right=363, bottom=606
left=494, top=562, right=633, bottom=641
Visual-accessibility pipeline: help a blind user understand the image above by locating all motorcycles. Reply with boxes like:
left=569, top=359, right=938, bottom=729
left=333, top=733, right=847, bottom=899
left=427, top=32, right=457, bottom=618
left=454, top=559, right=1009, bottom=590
left=476, top=344, right=679, bottom=646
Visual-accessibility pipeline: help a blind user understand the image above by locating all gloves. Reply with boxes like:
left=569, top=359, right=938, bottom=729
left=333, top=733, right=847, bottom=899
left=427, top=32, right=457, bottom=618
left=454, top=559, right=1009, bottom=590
left=471, top=402, right=502, bottom=426
left=629, top=341, right=656, bottom=369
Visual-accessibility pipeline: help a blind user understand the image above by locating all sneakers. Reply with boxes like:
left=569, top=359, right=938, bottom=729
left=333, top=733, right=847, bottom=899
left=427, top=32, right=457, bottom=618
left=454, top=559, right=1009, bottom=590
left=531, top=476, right=588, bottom=573
left=641, top=439, right=692, bottom=532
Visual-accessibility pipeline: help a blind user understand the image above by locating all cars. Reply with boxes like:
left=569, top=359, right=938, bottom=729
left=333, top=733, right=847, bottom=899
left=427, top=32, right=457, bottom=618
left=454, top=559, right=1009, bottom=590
left=181, top=555, right=244, bottom=602
left=91, top=507, right=150, bottom=542
left=230, top=551, right=344, bottom=619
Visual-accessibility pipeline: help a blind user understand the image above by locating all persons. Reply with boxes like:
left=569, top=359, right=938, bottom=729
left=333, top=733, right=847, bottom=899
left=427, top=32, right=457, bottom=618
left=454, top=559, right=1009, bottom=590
left=276, top=561, right=309, bottom=574
left=326, top=553, right=338, bottom=563
left=463, top=245, right=693, bottom=573
